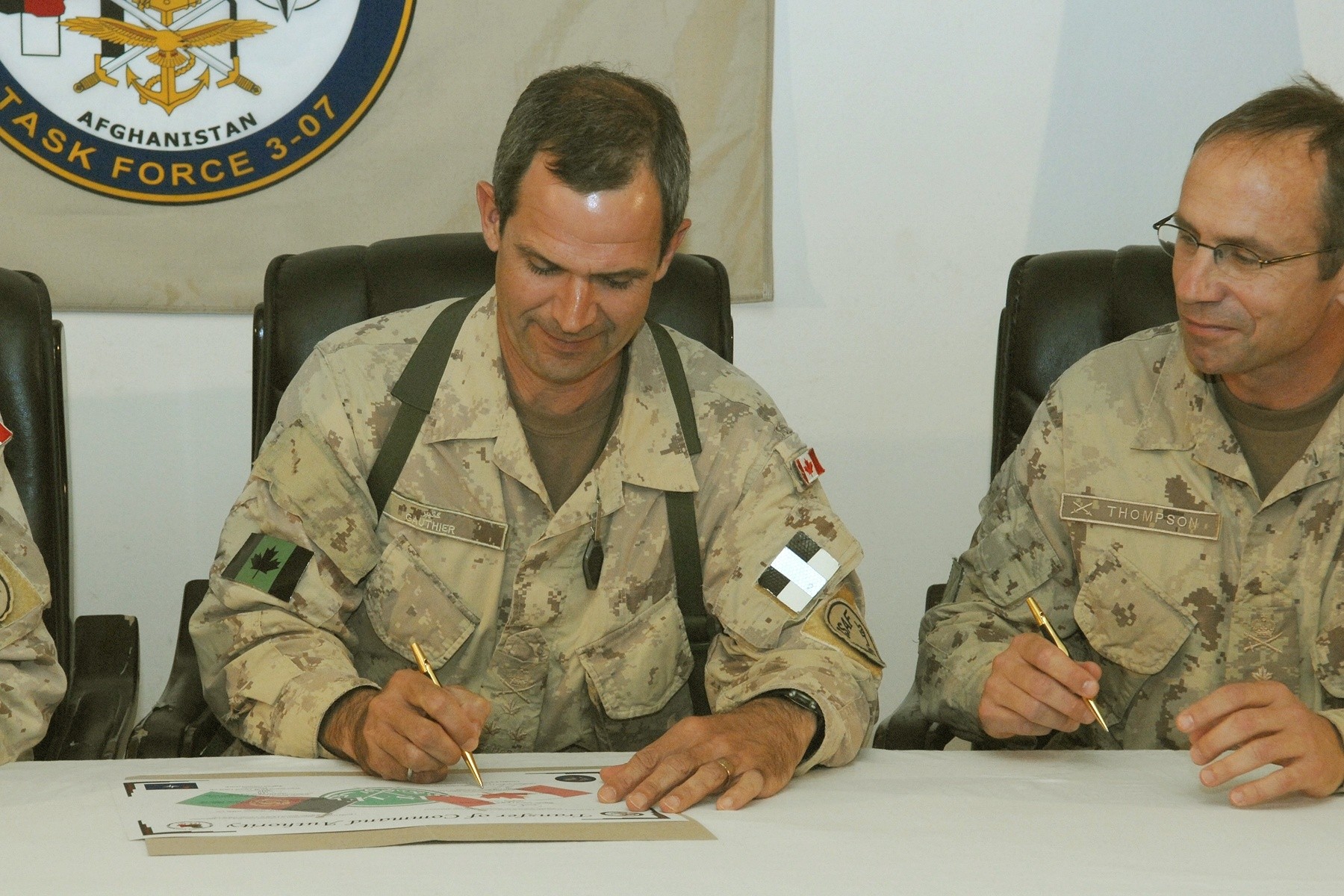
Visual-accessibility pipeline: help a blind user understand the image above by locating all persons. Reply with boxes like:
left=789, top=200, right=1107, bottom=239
left=191, top=66, right=884, bottom=811
left=914, top=74, right=1344, bottom=807
left=0, top=414, right=68, bottom=766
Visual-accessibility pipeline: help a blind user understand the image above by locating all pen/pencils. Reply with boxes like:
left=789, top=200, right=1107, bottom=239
left=411, top=641, right=484, bottom=789
left=1026, top=596, right=1110, bottom=734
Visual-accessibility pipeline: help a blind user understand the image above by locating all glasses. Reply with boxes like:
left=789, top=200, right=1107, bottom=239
left=1152, top=210, right=1343, bottom=282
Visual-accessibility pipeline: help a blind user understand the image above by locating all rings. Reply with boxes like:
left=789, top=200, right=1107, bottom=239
left=407, top=768, right=413, bottom=782
left=717, top=757, right=734, bottom=790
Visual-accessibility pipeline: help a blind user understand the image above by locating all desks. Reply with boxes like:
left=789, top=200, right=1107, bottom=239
left=0, top=752, right=1344, bottom=896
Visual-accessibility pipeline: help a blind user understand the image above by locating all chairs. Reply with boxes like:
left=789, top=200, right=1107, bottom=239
left=872, top=246, right=1180, bottom=752
left=124, top=230, right=736, bottom=760
left=0, top=267, right=141, bottom=761
